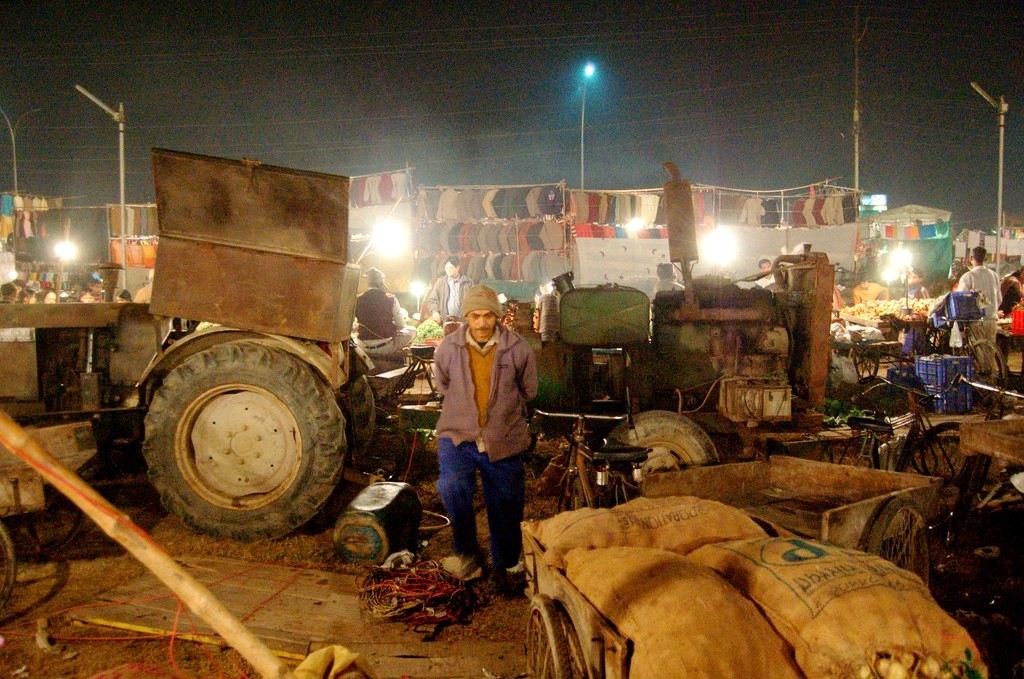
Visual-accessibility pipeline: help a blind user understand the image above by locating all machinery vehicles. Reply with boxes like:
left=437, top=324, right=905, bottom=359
left=524, top=161, right=837, bottom=477
left=0, top=145, right=377, bottom=540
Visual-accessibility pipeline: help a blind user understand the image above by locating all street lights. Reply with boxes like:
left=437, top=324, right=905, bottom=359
left=580, top=63, right=596, bottom=190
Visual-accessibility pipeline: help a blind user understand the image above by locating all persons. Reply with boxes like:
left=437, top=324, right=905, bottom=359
left=999, top=267, right=1024, bottom=313
left=852, top=274, right=891, bottom=303
left=655, top=260, right=689, bottom=292
left=0, top=267, right=154, bottom=305
left=427, top=256, right=473, bottom=325
left=754, top=258, right=778, bottom=288
left=956, top=247, right=1004, bottom=376
left=434, top=286, right=538, bottom=593
left=356, top=267, right=419, bottom=354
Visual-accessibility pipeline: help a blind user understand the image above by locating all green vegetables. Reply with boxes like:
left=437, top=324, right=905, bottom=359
left=415, top=319, right=443, bottom=342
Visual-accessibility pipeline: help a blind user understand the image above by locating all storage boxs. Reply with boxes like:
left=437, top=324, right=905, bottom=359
left=886, top=355, right=973, bottom=412
left=933, top=292, right=984, bottom=329
left=902, top=330, right=926, bottom=354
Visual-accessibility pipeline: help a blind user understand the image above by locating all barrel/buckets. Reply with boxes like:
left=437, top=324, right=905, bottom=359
left=333, top=482, right=422, bottom=568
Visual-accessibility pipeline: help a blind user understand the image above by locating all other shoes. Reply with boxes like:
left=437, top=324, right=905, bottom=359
left=499, top=567, right=516, bottom=591
left=460, top=548, right=485, bottom=576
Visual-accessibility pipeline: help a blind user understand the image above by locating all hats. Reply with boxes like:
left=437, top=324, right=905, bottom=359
left=366, top=269, right=384, bottom=284
left=462, top=285, right=500, bottom=318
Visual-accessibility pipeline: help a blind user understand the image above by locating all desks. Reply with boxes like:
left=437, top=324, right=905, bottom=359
left=840, top=312, right=1011, bottom=327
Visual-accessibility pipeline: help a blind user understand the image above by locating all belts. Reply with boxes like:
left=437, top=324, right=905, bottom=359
left=365, top=339, right=393, bottom=348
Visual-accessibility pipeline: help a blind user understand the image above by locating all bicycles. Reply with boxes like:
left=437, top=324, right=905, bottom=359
left=522, top=405, right=651, bottom=679
left=926, top=317, right=1006, bottom=388
left=846, top=327, right=882, bottom=377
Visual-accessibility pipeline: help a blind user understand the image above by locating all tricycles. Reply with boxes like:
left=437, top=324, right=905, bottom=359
left=643, top=378, right=967, bottom=590
